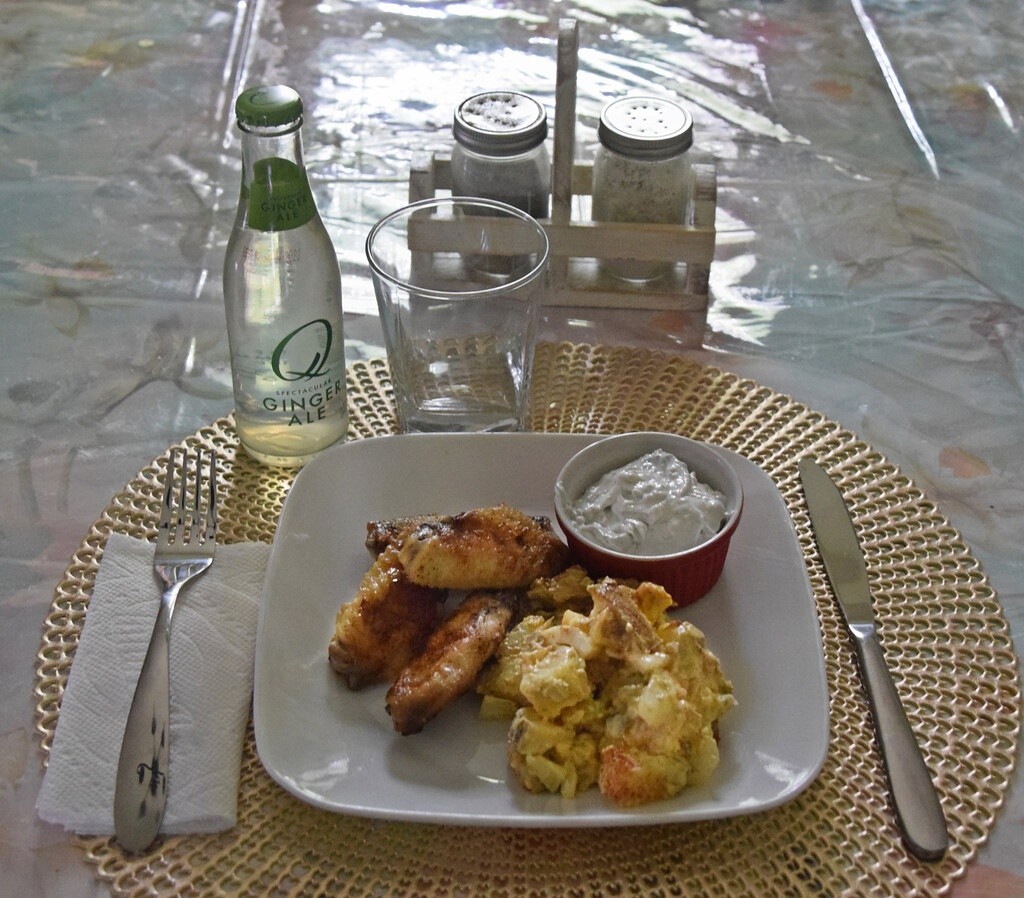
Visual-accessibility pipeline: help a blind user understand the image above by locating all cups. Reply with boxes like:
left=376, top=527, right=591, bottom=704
left=364, top=196, right=551, bottom=435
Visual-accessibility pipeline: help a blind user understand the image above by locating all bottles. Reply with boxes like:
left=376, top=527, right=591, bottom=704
left=223, top=83, right=349, bottom=468
left=450, top=90, right=552, bottom=279
left=591, top=96, right=695, bottom=283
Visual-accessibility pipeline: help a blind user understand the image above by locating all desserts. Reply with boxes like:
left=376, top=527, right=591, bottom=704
left=553, top=431, right=744, bottom=612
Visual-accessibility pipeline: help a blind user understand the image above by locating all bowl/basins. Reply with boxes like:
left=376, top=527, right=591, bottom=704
left=553, top=431, right=745, bottom=612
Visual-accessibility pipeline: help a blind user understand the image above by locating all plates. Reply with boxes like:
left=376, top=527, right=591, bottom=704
left=251, top=432, right=831, bottom=828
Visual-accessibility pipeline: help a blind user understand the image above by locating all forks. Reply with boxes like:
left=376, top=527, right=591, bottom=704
left=115, top=450, right=218, bottom=853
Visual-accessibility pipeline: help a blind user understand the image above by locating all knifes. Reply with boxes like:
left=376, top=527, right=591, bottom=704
left=798, top=459, right=949, bottom=861
